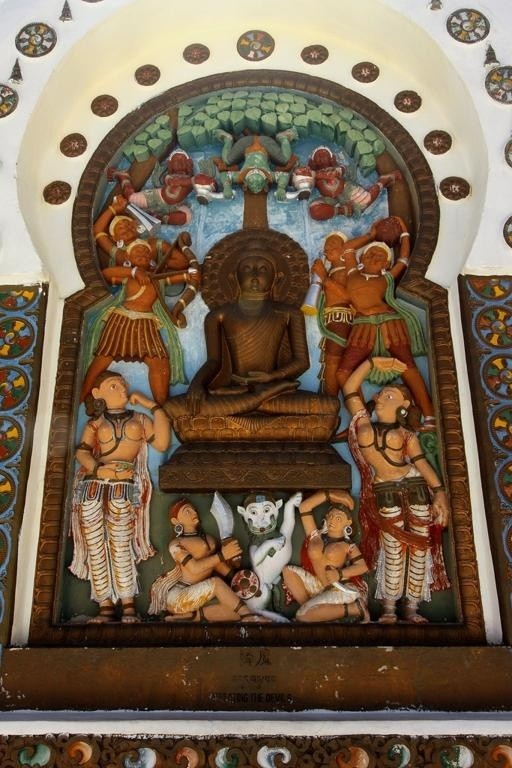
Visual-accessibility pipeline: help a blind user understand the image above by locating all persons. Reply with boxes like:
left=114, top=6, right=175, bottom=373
left=56, top=127, right=453, bottom=633
